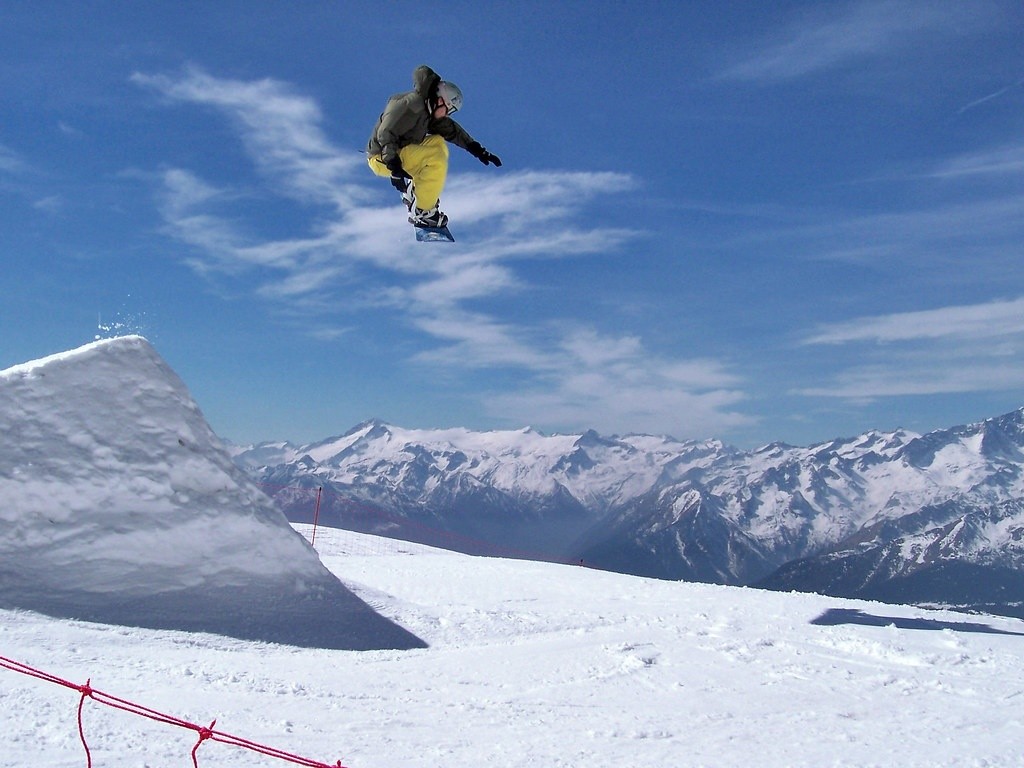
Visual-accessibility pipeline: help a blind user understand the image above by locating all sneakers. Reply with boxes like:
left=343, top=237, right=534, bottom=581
left=408, top=198, right=448, bottom=227
left=402, top=178, right=416, bottom=209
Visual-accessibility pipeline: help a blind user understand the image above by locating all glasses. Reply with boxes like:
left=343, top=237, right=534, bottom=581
left=446, top=106, right=456, bottom=117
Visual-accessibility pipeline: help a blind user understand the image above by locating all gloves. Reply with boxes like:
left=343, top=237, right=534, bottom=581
left=387, top=153, right=413, bottom=193
left=466, top=140, right=502, bottom=167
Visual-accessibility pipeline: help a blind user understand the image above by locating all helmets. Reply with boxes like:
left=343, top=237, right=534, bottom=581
left=437, top=80, right=463, bottom=116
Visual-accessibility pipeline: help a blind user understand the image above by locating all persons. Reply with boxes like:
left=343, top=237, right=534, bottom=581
left=364, top=65, right=503, bottom=227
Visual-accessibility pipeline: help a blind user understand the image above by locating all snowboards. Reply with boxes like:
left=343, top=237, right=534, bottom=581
left=400, top=190, right=455, bottom=243
